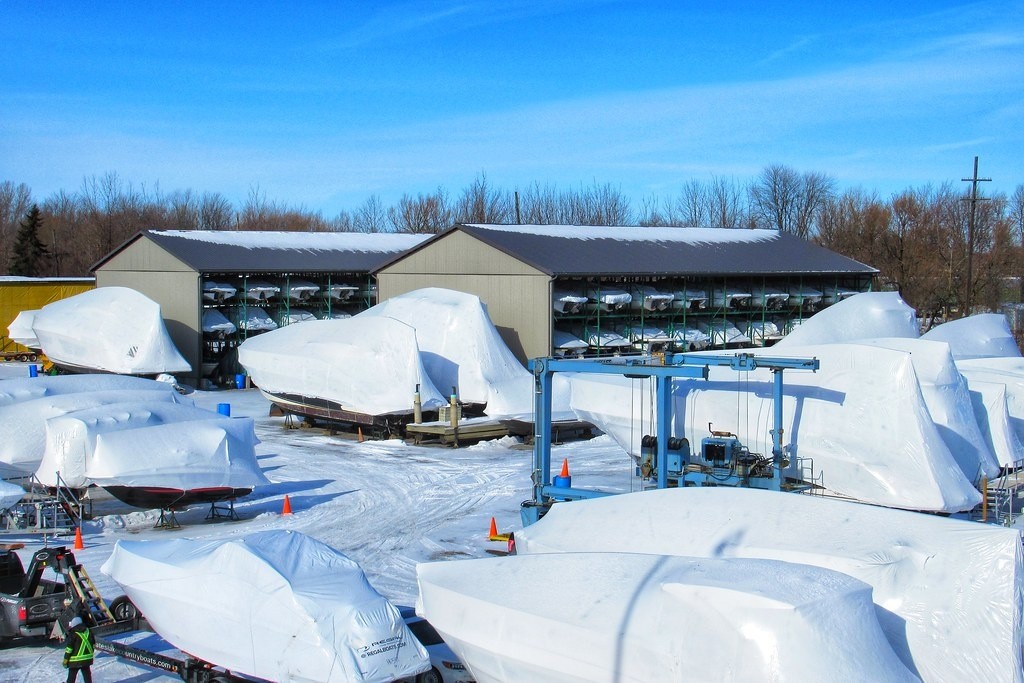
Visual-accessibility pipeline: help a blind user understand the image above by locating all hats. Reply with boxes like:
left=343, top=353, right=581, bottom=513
left=71, top=617, right=83, bottom=628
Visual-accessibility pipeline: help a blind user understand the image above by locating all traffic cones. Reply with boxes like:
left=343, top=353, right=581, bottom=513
left=74, top=527, right=86, bottom=550
left=358, top=427, right=365, bottom=442
left=488, top=517, right=499, bottom=536
left=557, top=458, right=570, bottom=477
left=281, top=494, right=293, bottom=515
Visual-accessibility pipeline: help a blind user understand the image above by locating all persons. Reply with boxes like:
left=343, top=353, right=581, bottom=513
left=62, top=618, right=95, bottom=683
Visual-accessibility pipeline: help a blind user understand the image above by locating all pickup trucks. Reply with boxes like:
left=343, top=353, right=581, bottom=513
left=1, top=543, right=92, bottom=649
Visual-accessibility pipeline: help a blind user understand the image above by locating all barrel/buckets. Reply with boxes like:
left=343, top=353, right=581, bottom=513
left=29, top=365, right=38, bottom=377
left=236, top=375, right=245, bottom=389
left=200, top=379, right=209, bottom=391
left=50, top=370, right=57, bottom=377
left=554, top=474, right=571, bottom=502
left=217, top=403, right=230, bottom=418
